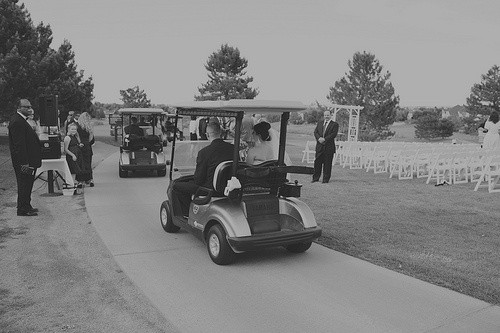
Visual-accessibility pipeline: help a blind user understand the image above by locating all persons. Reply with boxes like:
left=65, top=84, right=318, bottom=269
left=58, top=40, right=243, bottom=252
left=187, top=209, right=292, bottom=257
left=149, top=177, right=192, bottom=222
left=77, top=112, right=95, bottom=187
left=64, top=122, right=84, bottom=195
left=9, top=95, right=41, bottom=216
left=62, top=109, right=273, bottom=150
left=173, top=121, right=242, bottom=223
left=58, top=109, right=61, bottom=133
left=245, top=121, right=291, bottom=182
left=311, top=109, right=339, bottom=184
left=25, top=108, right=38, bottom=133
left=477, top=112, right=500, bottom=163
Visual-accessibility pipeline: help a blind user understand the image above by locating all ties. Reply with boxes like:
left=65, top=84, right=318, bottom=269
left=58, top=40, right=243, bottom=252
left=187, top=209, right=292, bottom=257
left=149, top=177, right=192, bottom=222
left=323, top=122, right=328, bottom=133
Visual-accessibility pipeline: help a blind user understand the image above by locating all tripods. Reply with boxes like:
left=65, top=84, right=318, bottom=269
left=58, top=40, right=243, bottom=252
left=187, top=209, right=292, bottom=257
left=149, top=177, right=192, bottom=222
left=32, top=126, right=67, bottom=189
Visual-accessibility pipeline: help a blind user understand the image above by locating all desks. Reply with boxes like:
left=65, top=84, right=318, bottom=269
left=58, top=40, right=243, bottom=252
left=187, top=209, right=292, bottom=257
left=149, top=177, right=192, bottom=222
left=34, top=154, right=74, bottom=197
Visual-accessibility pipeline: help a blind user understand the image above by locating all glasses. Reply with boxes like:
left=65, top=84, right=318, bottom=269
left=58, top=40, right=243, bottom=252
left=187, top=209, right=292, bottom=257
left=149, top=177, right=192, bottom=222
left=20, top=105, right=32, bottom=109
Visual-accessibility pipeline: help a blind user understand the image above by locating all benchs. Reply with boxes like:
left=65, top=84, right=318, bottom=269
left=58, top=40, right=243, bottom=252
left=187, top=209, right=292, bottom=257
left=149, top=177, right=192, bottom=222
left=123, top=134, right=161, bottom=154
left=213, top=159, right=288, bottom=206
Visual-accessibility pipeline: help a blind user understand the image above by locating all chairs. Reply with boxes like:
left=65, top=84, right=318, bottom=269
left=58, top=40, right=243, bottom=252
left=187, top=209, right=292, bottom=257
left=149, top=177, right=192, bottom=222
left=301, top=138, right=500, bottom=194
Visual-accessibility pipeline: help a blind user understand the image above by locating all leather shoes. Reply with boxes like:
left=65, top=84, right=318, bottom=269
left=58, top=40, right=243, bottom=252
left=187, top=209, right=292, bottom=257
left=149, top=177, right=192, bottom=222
left=30, top=208, right=39, bottom=212
left=17, top=210, right=38, bottom=216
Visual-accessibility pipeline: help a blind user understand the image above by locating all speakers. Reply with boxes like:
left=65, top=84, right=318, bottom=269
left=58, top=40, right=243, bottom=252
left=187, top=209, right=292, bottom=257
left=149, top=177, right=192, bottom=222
left=39, top=94, right=58, bottom=126
